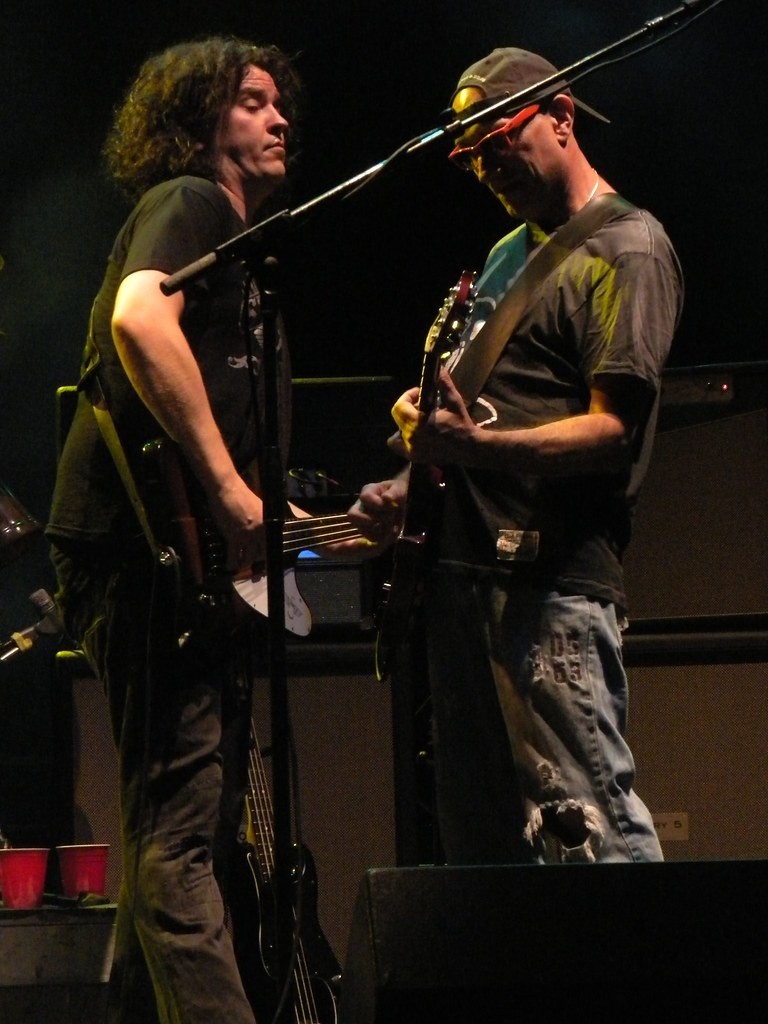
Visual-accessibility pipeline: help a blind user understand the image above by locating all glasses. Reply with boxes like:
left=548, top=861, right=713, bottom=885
left=448, top=98, right=544, bottom=172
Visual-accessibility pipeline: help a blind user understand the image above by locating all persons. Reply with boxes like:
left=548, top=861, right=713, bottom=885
left=48, top=42, right=390, bottom=1024
left=350, top=46, right=683, bottom=867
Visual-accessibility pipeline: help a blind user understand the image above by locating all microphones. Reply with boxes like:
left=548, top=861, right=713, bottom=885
left=28, top=588, right=75, bottom=647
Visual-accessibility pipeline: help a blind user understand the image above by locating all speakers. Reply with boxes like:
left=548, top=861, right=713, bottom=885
left=0, top=904, right=117, bottom=1024
left=336, top=858, right=768, bottom=1024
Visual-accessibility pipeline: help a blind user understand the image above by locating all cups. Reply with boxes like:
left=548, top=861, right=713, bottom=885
left=55, top=844, right=110, bottom=898
left=0, top=847, right=51, bottom=910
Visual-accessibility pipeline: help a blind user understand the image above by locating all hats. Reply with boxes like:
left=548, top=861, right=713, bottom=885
left=448, top=47, right=611, bottom=124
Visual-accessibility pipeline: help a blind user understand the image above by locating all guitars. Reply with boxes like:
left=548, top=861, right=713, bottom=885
left=167, top=443, right=365, bottom=641
left=234, top=713, right=342, bottom=1023
left=381, top=269, right=480, bottom=637
